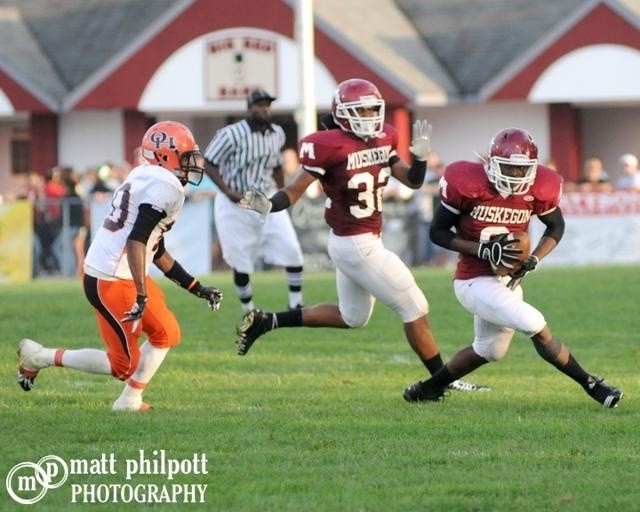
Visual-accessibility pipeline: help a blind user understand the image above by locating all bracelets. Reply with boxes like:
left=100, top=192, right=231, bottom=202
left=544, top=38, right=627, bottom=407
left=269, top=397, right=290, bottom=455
left=164, top=260, right=194, bottom=289
left=407, top=159, right=426, bottom=185
left=269, top=191, right=290, bottom=212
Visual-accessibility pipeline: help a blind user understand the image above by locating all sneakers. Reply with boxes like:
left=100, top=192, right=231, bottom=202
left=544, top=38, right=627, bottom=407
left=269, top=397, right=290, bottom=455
left=402, top=380, right=449, bottom=405
left=112, top=402, right=155, bottom=413
left=584, top=375, right=624, bottom=409
left=447, top=380, right=493, bottom=393
left=16, top=338, right=43, bottom=392
left=234, top=308, right=266, bottom=356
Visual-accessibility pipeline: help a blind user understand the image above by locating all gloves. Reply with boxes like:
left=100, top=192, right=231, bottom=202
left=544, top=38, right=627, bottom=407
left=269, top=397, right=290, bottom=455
left=191, top=282, right=223, bottom=311
left=478, top=232, right=523, bottom=271
left=408, top=119, right=433, bottom=161
left=237, top=187, right=274, bottom=223
left=119, top=294, right=149, bottom=334
left=506, top=255, right=539, bottom=291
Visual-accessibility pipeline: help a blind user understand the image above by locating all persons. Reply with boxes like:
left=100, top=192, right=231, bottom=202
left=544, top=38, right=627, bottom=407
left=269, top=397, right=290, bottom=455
left=17, top=121, right=223, bottom=413
left=381, top=154, right=446, bottom=266
left=608, top=154, right=640, bottom=192
left=0, top=156, right=129, bottom=281
left=403, top=127, right=624, bottom=408
left=204, top=89, right=304, bottom=318
left=183, top=158, right=218, bottom=201
left=577, top=157, right=614, bottom=192
left=235, top=79, right=491, bottom=393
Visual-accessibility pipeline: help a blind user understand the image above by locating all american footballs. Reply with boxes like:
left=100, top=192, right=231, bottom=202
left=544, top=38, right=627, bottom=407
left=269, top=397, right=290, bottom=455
left=491, top=231, right=531, bottom=275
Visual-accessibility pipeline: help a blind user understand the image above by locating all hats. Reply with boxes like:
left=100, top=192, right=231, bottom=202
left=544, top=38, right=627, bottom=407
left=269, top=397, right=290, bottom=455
left=247, top=89, right=277, bottom=107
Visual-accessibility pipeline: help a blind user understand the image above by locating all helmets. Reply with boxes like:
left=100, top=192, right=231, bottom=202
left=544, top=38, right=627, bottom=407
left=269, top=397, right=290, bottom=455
left=483, top=127, right=539, bottom=195
left=140, top=121, right=205, bottom=186
left=331, top=78, right=386, bottom=139
